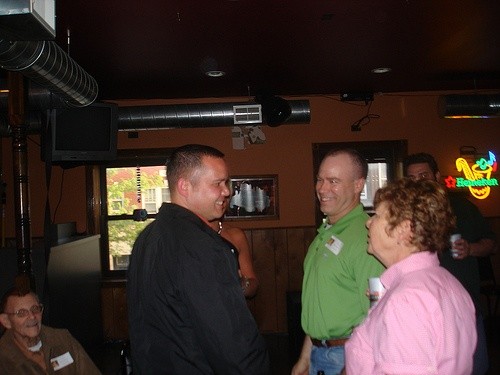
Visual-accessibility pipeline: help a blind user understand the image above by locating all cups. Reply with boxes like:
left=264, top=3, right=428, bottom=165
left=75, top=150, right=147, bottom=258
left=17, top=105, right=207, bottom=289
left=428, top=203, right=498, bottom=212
left=449, top=234, right=462, bottom=257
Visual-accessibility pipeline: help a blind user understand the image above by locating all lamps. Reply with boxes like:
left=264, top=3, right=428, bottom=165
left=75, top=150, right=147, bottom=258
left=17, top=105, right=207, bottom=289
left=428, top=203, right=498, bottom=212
left=205, top=71, right=225, bottom=77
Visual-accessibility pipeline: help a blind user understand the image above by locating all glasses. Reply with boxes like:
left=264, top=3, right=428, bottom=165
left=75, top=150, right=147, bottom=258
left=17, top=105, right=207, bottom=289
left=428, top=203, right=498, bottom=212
left=3, top=304, right=43, bottom=317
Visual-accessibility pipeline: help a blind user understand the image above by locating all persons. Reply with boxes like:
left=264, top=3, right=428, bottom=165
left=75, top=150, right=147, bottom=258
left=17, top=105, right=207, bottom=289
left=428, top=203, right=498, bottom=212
left=290, top=145, right=388, bottom=375
left=405, top=153, right=496, bottom=375
left=1, top=279, right=101, bottom=374
left=124, top=143, right=273, bottom=375
left=208, top=220, right=258, bottom=298
left=343, top=174, right=478, bottom=375
left=52, top=361, right=59, bottom=367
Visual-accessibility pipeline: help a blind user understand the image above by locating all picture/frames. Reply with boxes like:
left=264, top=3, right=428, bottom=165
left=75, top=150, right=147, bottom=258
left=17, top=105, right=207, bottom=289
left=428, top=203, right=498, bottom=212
left=222, top=174, right=279, bottom=222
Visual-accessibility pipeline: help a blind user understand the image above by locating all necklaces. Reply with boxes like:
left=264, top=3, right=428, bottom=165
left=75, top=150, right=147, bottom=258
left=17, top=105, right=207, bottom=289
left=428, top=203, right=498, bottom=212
left=218, top=222, right=222, bottom=235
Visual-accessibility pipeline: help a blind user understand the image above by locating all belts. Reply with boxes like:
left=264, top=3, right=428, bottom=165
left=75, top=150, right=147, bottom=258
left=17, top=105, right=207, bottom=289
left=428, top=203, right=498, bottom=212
left=310, top=337, right=349, bottom=347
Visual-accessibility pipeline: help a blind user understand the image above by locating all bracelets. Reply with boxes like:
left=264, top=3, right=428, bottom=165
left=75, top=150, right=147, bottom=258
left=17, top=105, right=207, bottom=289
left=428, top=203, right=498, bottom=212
left=242, top=275, right=250, bottom=291
